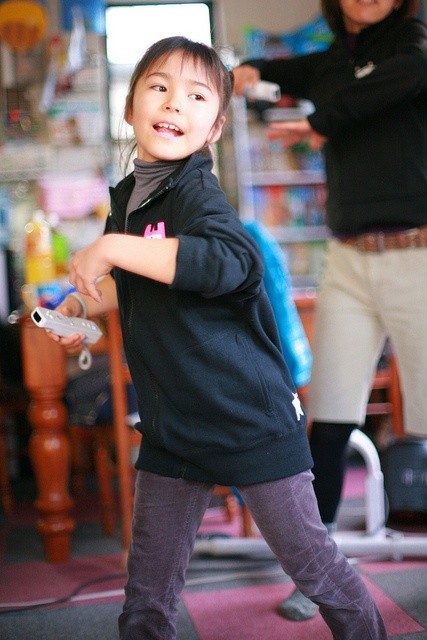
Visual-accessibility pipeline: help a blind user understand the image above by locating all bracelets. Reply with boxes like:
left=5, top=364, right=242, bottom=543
left=65, top=291, right=86, bottom=320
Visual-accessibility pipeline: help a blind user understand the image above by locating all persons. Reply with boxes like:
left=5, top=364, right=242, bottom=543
left=44, top=36, right=387, bottom=640
left=231, top=0, right=426, bottom=621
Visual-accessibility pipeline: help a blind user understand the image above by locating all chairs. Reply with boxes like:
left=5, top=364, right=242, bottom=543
left=92, top=306, right=253, bottom=550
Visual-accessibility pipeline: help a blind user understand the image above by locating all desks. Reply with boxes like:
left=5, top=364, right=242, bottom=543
left=15, top=292, right=317, bottom=561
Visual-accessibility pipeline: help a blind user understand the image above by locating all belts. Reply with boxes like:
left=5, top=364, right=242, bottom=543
left=331, top=222, right=426, bottom=254
